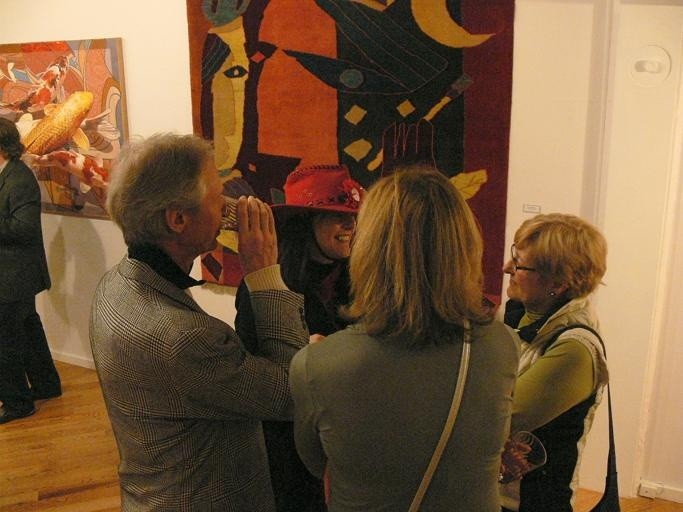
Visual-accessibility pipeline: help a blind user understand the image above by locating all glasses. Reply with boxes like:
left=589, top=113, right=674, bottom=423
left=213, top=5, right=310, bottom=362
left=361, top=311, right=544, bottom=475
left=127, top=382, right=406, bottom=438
left=509, top=239, right=544, bottom=275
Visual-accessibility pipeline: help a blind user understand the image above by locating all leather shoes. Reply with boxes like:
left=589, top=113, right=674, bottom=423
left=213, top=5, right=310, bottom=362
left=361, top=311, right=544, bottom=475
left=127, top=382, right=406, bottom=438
left=0, top=402, right=35, bottom=424
left=27, top=386, right=62, bottom=402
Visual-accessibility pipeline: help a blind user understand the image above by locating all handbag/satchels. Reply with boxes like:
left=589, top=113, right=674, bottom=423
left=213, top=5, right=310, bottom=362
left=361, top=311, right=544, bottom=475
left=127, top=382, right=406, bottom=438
left=587, top=453, right=628, bottom=512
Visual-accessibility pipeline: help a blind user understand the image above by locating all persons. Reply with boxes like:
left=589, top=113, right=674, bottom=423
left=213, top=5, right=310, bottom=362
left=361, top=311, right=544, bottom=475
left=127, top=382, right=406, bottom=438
left=0, top=112, right=65, bottom=425
left=487, top=209, right=610, bottom=511
left=85, top=129, right=310, bottom=510
left=287, top=162, right=520, bottom=508
left=224, top=157, right=361, bottom=511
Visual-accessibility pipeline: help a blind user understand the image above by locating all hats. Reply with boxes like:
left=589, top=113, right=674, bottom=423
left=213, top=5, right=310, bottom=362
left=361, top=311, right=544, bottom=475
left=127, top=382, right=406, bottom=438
left=269, top=163, right=366, bottom=215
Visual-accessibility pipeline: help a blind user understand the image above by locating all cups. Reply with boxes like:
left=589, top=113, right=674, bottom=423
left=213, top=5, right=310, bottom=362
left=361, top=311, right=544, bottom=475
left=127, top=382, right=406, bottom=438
left=221, top=195, right=251, bottom=232
left=499, top=431, right=547, bottom=485
